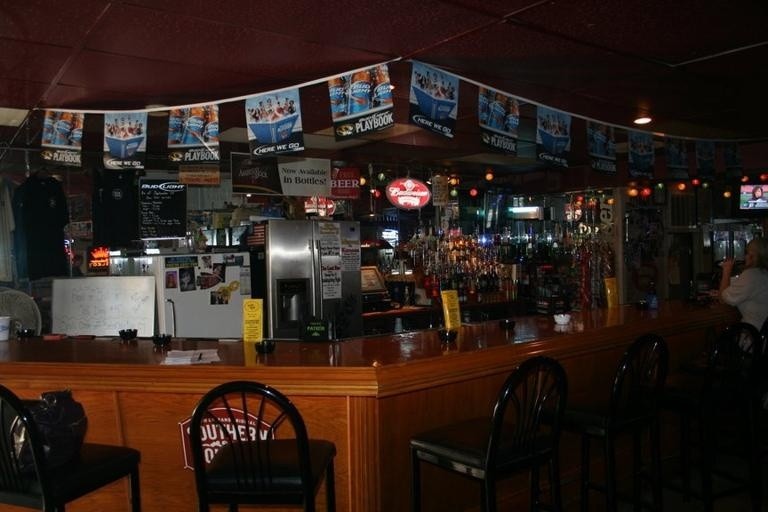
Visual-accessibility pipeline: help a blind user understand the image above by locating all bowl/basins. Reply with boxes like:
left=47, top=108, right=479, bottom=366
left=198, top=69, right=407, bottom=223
left=499, top=320, right=517, bottom=330
left=554, top=313, right=572, bottom=324
left=635, top=299, right=649, bottom=310
left=255, top=341, right=276, bottom=356
left=17, top=329, right=35, bottom=339
left=119, top=329, right=138, bottom=340
left=151, top=334, right=173, bottom=345
left=553, top=323, right=571, bottom=333
left=437, top=328, right=458, bottom=343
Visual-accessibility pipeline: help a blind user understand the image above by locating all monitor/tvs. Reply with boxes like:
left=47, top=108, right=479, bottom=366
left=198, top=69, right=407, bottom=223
left=360, top=266, right=388, bottom=295
left=738, top=182, right=768, bottom=212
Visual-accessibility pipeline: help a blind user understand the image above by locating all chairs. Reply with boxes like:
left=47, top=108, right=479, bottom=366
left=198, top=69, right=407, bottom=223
left=1, top=382, right=146, bottom=512
left=557, top=332, right=677, bottom=510
left=655, top=321, right=766, bottom=512
left=190, top=378, right=339, bottom=511
left=407, top=355, right=573, bottom=512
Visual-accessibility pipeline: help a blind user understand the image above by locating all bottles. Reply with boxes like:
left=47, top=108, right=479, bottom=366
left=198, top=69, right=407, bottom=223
left=647, top=280, right=658, bottom=311
left=368, top=215, right=399, bottom=230
left=40, top=109, right=82, bottom=148
left=581, top=194, right=602, bottom=221
left=168, top=105, right=218, bottom=146
left=246, top=94, right=298, bottom=121
left=187, top=201, right=234, bottom=250
left=104, top=114, right=146, bottom=139
left=688, top=279, right=696, bottom=299
left=327, top=66, right=393, bottom=121
left=379, top=220, right=593, bottom=316
left=415, top=64, right=686, bottom=165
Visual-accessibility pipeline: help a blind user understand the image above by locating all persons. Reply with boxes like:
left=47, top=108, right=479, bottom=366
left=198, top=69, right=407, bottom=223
left=718, top=239, right=768, bottom=353
left=745, top=186, right=768, bottom=208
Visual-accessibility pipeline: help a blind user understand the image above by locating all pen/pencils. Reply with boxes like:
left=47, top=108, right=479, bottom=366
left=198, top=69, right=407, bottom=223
left=198, top=352, right=203, bottom=361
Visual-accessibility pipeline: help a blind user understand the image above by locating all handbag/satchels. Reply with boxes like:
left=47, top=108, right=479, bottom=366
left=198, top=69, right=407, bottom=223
left=8, top=388, right=88, bottom=479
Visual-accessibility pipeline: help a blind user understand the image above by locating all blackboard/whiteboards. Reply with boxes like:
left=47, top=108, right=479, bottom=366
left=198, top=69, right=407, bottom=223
left=52, top=275, right=156, bottom=337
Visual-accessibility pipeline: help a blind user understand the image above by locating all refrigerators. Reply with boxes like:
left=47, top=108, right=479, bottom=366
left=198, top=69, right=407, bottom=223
left=239, top=216, right=364, bottom=341
left=110, top=251, right=252, bottom=340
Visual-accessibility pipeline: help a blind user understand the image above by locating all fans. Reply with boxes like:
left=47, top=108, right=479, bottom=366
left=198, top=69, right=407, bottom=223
left=0, top=288, right=44, bottom=336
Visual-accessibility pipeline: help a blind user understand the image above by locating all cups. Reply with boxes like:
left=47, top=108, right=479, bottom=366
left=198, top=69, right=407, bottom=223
left=0, top=316, right=11, bottom=342
